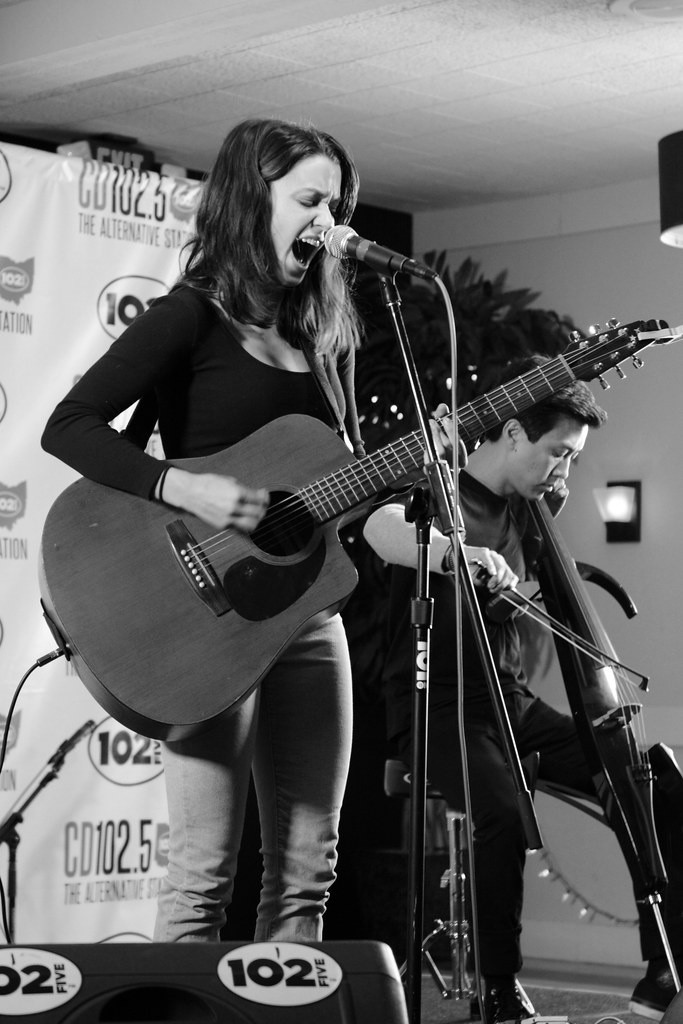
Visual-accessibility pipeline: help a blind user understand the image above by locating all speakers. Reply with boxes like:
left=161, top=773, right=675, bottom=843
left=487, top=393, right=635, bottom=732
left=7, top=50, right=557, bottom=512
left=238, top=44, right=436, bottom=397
left=0, top=939, right=410, bottom=1024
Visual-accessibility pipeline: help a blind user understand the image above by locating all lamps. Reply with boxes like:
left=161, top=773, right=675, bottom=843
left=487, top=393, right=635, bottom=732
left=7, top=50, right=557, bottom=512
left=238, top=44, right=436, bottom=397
left=591, top=480, right=642, bottom=546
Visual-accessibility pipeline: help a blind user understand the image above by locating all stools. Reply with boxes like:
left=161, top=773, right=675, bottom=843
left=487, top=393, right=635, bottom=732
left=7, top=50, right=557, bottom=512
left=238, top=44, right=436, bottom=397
left=383, top=758, right=543, bottom=1018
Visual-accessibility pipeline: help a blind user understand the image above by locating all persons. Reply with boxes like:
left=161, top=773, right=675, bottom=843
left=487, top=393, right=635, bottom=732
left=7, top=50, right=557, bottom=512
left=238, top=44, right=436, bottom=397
left=40, top=118, right=469, bottom=947
left=363, top=351, right=683, bottom=1023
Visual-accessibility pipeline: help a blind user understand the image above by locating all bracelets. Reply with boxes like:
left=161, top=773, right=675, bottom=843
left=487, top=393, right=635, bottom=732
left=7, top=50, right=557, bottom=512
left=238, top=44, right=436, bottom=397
left=159, top=465, right=172, bottom=502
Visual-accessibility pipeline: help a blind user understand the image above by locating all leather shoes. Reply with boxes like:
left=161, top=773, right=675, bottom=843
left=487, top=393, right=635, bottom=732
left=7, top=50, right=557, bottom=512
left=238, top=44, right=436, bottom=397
left=468, top=975, right=537, bottom=1024
left=627, top=968, right=682, bottom=1022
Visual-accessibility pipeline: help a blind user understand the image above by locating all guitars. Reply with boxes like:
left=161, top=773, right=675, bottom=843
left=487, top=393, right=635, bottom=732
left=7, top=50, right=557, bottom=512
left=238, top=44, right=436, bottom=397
left=36, top=315, right=682, bottom=743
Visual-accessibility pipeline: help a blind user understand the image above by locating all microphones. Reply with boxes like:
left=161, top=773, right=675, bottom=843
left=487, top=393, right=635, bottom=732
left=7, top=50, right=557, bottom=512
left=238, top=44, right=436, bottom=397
left=324, top=225, right=440, bottom=280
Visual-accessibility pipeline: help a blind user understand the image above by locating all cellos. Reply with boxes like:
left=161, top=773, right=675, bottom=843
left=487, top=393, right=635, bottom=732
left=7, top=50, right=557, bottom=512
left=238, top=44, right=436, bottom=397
left=513, top=487, right=682, bottom=992
